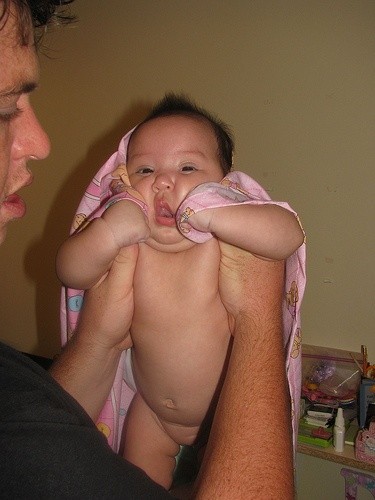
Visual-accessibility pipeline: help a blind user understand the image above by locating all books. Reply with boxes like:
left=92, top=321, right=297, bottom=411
left=304, top=402, right=338, bottom=429
left=345, top=424, right=360, bottom=447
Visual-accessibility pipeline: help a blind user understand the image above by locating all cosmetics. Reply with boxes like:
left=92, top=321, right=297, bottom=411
left=334, top=409, right=346, bottom=453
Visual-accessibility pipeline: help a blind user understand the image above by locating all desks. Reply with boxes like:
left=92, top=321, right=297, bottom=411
left=294, top=423, right=375, bottom=475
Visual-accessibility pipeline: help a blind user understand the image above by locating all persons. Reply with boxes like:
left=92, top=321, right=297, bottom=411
left=0, top=0, right=300, bottom=500
left=53, top=90, right=306, bottom=500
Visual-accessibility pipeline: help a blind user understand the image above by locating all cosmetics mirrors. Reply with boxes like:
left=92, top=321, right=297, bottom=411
left=338, top=395, right=356, bottom=405
